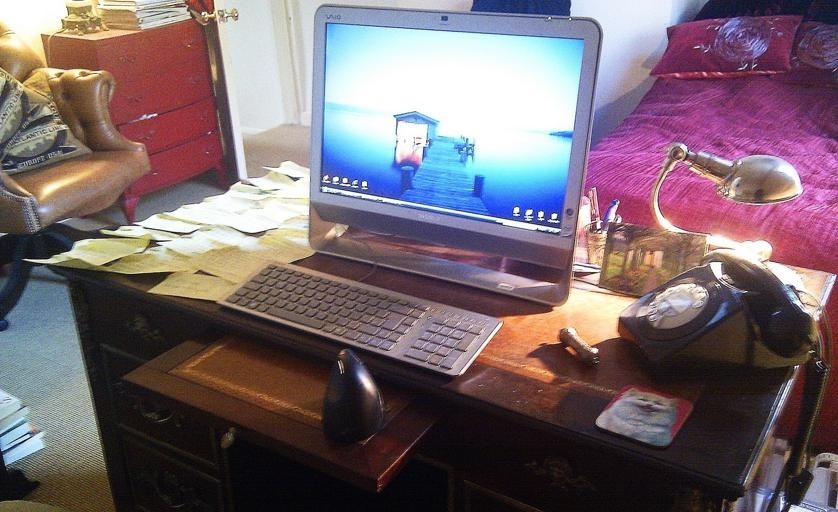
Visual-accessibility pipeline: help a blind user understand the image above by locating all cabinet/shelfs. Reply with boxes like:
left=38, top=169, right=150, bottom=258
left=41, top=20, right=225, bottom=226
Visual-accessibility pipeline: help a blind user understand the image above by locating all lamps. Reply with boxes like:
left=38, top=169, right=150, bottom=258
left=649, top=142, right=805, bottom=263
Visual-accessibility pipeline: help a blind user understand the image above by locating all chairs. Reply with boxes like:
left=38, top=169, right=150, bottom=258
left=0, top=21, right=152, bottom=333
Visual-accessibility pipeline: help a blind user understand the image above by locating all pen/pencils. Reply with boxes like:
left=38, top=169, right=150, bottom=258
left=589, top=187, right=622, bottom=233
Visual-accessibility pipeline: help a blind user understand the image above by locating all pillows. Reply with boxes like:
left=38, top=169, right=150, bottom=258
left=649, top=14, right=838, bottom=81
left=0, top=67, right=92, bottom=176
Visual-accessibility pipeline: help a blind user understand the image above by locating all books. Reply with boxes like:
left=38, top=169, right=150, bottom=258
left=95, top=0, right=192, bottom=32
left=0, top=389, right=47, bottom=466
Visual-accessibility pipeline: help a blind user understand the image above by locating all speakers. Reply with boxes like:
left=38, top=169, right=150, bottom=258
left=322, top=348, right=385, bottom=443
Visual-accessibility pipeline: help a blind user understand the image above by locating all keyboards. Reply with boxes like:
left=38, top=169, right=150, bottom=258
left=214, top=257, right=504, bottom=378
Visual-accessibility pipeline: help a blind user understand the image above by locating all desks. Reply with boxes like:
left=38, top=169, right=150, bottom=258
left=52, top=164, right=837, bottom=512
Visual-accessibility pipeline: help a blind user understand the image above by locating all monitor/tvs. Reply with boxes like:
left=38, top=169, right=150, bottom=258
left=309, top=6, right=603, bottom=308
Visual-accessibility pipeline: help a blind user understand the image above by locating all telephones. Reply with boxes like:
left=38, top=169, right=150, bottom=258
left=618, top=249, right=817, bottom=376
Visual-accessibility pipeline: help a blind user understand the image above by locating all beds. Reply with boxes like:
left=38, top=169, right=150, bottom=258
left=585, top=1, right=838, bottom=448
